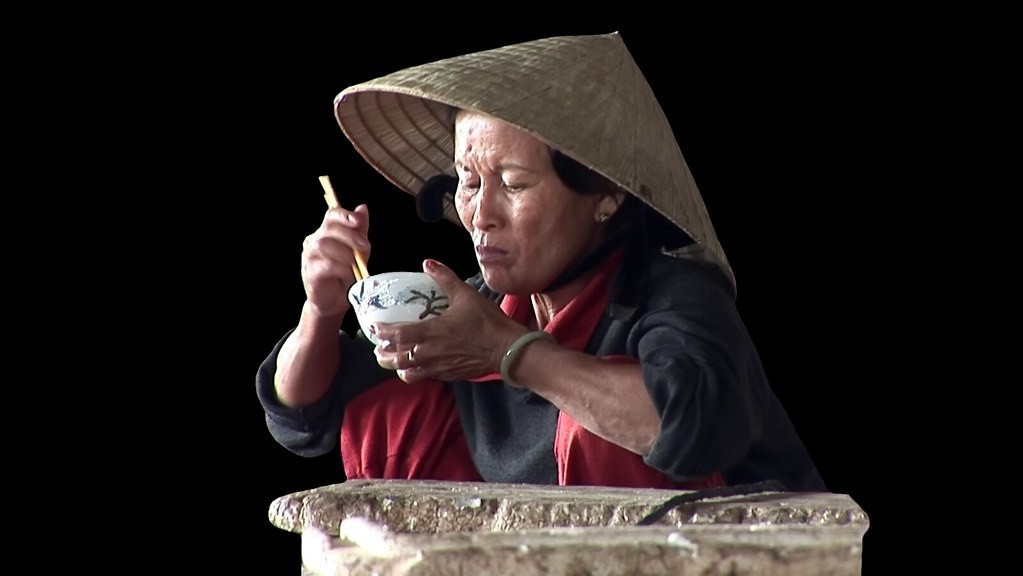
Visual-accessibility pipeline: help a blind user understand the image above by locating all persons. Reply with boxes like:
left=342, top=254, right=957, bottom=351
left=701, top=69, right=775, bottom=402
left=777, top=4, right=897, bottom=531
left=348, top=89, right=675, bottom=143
left=255, top=31, right=828, bottom=491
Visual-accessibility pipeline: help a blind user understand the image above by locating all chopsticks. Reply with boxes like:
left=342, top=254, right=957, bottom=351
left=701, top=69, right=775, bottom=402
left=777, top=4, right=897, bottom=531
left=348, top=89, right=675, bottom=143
left=320, top=175, right=370, bottom=279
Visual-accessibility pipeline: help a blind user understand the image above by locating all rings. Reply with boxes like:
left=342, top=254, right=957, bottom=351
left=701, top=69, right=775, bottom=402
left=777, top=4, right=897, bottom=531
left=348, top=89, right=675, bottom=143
left=409, top=344, right=418, bottom=365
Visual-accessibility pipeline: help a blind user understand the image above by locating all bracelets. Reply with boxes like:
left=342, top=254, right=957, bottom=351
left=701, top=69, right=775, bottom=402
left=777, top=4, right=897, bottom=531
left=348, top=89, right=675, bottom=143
left=500, top=332, right=555, bottom=388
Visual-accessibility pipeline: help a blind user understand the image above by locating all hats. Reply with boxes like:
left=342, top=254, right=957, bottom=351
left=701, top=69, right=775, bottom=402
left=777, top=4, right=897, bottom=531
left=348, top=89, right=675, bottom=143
left=333, top=30, right=739, bottom=298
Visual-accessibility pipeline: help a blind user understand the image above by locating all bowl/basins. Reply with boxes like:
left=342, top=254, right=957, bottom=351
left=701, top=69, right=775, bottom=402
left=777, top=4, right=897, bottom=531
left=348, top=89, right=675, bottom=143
left=347, top=271, right=449, bottom=354
